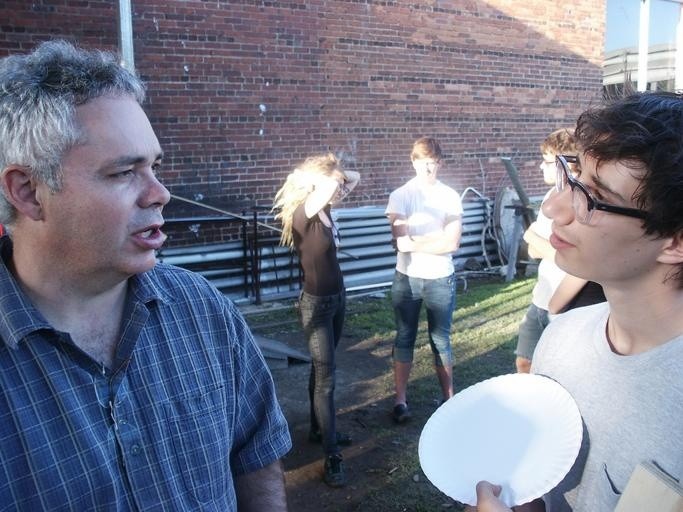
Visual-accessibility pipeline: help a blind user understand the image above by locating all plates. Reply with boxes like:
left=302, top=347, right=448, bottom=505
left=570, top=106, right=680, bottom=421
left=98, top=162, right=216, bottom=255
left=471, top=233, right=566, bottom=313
left=417, top=372, right=584, bottom=508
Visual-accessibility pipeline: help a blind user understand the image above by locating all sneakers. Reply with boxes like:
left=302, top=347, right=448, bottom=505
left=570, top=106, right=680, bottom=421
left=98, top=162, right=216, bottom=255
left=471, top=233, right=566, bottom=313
left=323, top=453, right=345, bottom=487
left=309, top=430, right=353, bottom=447
left=392, top=400, right=412, bottom=424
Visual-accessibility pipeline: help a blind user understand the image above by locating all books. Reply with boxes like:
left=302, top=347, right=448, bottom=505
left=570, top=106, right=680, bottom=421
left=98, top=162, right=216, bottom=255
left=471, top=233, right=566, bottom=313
left=611, top=456, right=683, bottom=512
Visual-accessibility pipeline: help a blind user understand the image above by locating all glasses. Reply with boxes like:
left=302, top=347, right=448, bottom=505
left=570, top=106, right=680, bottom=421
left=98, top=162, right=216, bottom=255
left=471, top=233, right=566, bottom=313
left=554, top=152, right=656, bottom=226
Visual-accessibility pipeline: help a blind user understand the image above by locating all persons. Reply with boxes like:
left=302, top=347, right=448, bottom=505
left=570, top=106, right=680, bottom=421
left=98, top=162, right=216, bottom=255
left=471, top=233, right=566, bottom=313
left=460, top=90, right=682, bottom=512
left=267, top=154, right=362, bottom=488
left=512, top=125, right=582, bottom=373
left=548, top=272, right=607, bottom=316
left=1, top=39, right=294, bottom=512
left=383, top=136, right=463, bottom=426
